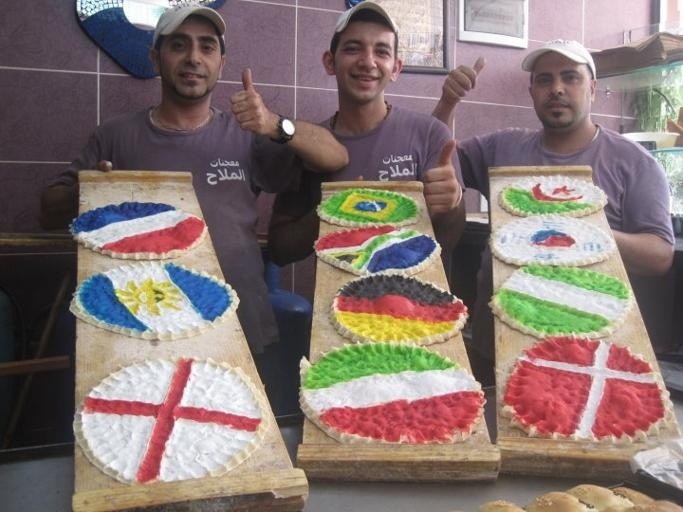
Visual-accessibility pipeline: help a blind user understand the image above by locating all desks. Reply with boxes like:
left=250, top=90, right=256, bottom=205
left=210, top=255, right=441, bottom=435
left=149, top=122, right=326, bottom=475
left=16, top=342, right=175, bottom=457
left=0, top=357, right=683, bottom=512
left=0, top=169, right=88, bottom=452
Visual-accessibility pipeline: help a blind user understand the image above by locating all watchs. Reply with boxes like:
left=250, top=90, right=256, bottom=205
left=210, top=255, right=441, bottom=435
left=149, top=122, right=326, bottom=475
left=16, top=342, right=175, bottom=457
left=271, top=114, right=295, bottom=144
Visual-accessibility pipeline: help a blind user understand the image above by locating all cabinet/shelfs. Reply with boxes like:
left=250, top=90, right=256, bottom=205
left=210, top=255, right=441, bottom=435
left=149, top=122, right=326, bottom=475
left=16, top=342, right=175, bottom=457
left=592, top=59, right=682, bottom=160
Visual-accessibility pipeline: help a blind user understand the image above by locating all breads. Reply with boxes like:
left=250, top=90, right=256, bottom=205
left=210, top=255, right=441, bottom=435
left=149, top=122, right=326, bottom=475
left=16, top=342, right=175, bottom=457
left=478, top=483, right=683, bottom=512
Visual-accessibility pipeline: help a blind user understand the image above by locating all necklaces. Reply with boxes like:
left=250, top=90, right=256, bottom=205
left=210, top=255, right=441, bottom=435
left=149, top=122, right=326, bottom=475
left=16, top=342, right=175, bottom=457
left=331, top=100, right=390, bottom=133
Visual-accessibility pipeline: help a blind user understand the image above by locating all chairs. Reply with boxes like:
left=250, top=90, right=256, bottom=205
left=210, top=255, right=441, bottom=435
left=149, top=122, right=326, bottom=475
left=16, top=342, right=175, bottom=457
left=256, top=247, right=311, bottom=387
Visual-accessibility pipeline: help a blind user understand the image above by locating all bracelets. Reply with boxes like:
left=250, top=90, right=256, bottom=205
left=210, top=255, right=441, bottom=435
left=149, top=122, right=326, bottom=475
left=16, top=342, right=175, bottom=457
left=455, top=184, right=462, bottom=207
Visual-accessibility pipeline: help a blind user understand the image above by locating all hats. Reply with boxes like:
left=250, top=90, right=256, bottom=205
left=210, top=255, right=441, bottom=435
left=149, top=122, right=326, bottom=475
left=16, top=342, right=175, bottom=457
left=522, top=38, right=597, bottom=82
left=335, top=1, right=400, bottom=38
left=153, top=5, right=226, bottom=48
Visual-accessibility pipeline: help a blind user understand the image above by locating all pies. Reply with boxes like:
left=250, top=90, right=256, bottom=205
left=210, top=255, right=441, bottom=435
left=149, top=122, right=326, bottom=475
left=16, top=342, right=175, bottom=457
left=314, top=224, right=442, bottom=277
left=330, top=272, right=470, bottom=347
left=298, top=339, right=488, bottom=445
left=500, top=334, right=674, bottom=444
left=497, top=175, right=608, bottom=218
left=490, top=214, right=615, bottom=267
left=72, top=357, right=271, bottom=486
left=70, top=261, right=240, bottom=341
left=67, top=201, right=210, bottom=261
left=316, top=188, right=420, bottom=226
left=487, top=264, right=633, bottom=339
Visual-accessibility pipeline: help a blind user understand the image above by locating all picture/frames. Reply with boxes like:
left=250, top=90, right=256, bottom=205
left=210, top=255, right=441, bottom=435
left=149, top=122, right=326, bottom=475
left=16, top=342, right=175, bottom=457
left=457, top=0, right=529, bottom=52
left=364, top=0, right=450, bottom=75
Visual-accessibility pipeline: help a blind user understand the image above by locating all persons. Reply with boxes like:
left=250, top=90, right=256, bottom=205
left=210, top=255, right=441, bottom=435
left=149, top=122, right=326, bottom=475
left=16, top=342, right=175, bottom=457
left=268, top=3, right=466, bottom=269
left=36, top=4, right=348, bottom=413
left=430, top=38, right=677, bottom=379
